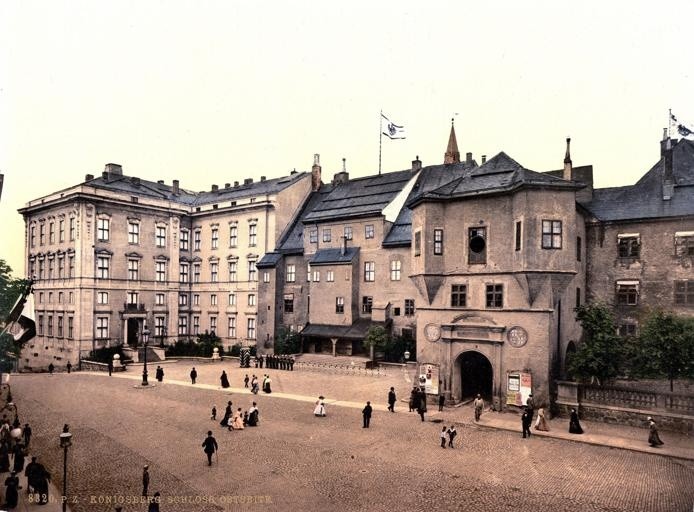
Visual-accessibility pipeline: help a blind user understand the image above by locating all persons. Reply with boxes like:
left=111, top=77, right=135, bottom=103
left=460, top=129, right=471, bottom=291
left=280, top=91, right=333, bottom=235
left=142, top=465, right=150, bottom=499
left=0, top=414, right=51, bottom=508
left=66, top=360, right=72, bottom=373
left=362, top=401, right=373, bottom=428
left=149, top=491, right=161, bottom=512
left=220, top=352, right=295, bottom=394
left=387, top=385, right=584, bottom=449
left=314, top=395, right=326, bottom=417
left=155, top=365, right=164, bottom=382
left=190, top=367, right=197, bottom=384
left=210, top=400, right=259, bottom=431
left=202, top=431, right=218, bottom=466
left=48, top=363, right=54, bottom=373
left=647, top=416, right=664, bottom=447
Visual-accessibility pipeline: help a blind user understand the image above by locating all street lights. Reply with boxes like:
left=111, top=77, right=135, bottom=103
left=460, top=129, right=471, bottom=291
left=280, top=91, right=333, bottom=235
left=55, top=424, right=72, bottom=512
left=403, top=349, right=411, bottom=365
left=140, top=325, right=150, bottom=386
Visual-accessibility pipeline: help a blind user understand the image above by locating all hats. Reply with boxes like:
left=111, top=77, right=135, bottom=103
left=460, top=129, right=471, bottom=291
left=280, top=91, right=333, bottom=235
left=477, top=393, right=481, bottom=396
left=529, top=394, right=532, bottom=395
left=143, top=464, right=149, bottom=468
left=571, top=407, right=576, bottom=412
left=319, top=395, right=325, bottom=398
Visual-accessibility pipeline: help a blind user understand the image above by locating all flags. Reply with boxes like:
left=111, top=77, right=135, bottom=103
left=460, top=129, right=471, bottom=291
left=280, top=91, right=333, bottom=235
left=381, top=113, right=406, bottom=139
